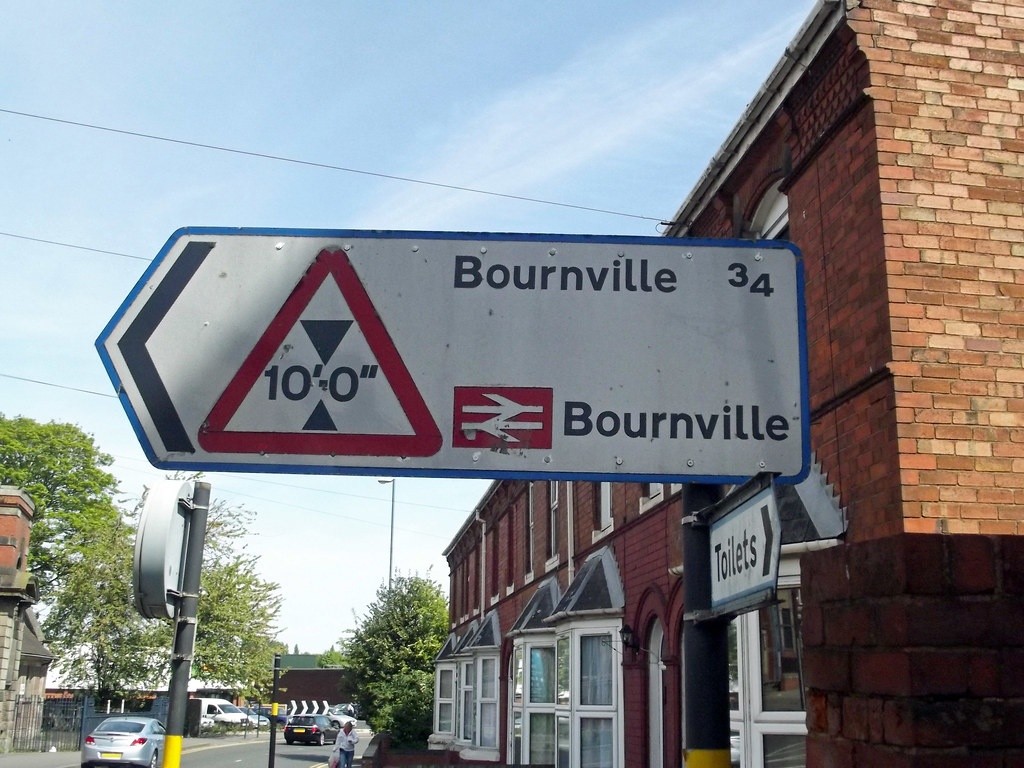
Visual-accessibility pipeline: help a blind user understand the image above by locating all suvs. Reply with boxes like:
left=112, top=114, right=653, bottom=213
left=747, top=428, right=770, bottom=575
left=254, top=707, right=287, bottom=729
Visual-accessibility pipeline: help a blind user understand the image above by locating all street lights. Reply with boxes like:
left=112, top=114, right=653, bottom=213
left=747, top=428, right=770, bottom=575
left=377, top=478, right=396, bottom=592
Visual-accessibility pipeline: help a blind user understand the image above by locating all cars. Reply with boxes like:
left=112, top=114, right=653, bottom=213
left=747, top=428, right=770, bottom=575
left=81, top=716, right=168, bottom=768
left=239, top=707, right=271, bottom=729
left=284, top=713, right=339, bottom=746
left=201, top=718, right=215, bottom=731
left=326, top=708, right=357, bottom=731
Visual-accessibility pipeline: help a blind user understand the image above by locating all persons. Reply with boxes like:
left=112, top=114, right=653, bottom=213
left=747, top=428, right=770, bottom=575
left=332, top=722, right=358, bottom=768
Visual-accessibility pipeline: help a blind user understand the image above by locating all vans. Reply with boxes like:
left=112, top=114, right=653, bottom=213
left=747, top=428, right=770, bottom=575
left=191, top=698, right=249, bottom=732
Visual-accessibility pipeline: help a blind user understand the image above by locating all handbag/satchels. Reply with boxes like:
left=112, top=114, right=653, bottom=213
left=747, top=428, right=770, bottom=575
left=328, top=751, right=340, bottom=768
